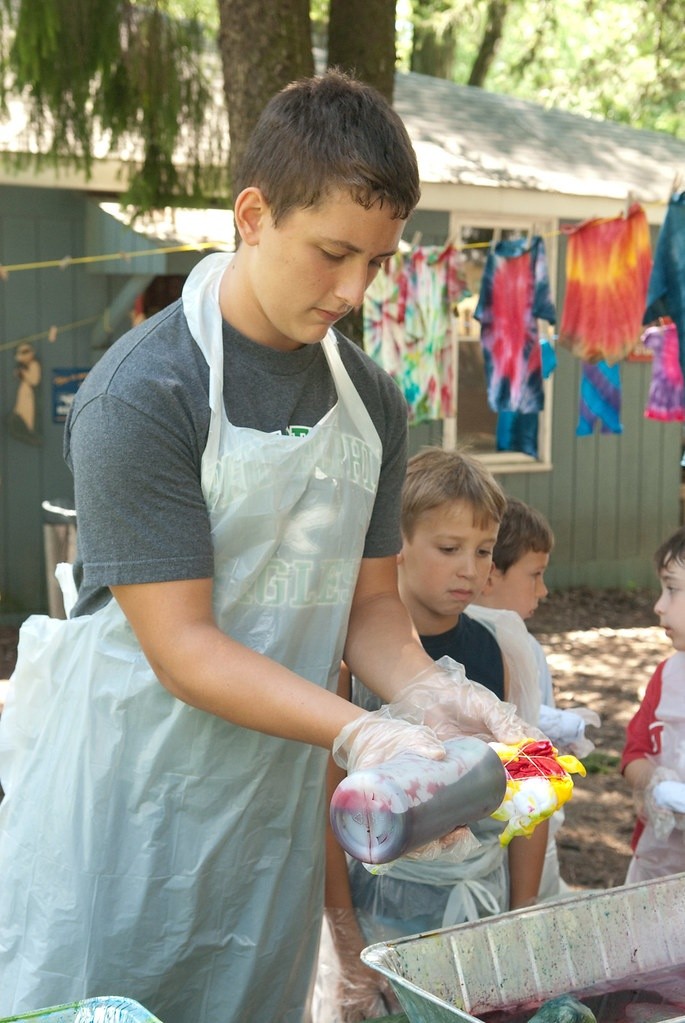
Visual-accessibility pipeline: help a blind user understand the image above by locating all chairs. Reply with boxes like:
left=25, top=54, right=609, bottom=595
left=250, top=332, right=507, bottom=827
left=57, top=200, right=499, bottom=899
left=40, top=500, right=81, bottom=619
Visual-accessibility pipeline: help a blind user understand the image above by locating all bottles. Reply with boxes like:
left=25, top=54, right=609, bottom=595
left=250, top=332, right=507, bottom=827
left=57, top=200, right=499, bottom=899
left=330, top=735, right=506, bottom=866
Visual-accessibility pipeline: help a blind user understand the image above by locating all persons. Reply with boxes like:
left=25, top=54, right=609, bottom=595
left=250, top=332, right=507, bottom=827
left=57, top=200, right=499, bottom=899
left=620, top=529, right=685, bottom=886
left=0, top=72, right=557, bottom=1023
left=324, top=448, right=601, bottom=1023
